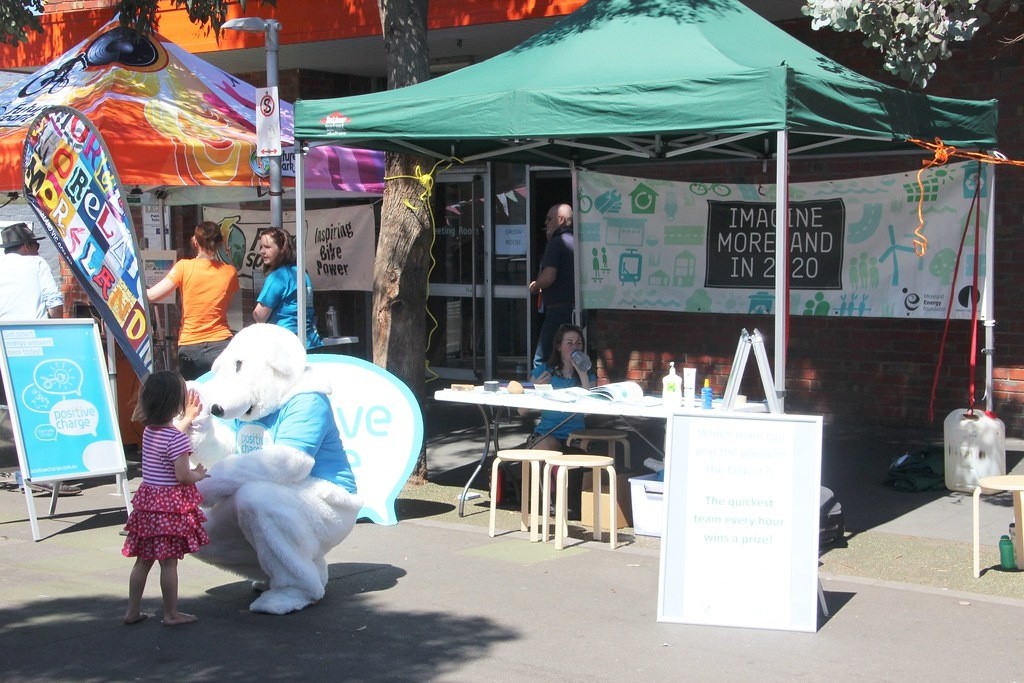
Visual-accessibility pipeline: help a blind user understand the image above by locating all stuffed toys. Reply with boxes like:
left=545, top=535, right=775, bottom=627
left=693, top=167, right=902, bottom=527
left=172, top=323, right=359, bottom=615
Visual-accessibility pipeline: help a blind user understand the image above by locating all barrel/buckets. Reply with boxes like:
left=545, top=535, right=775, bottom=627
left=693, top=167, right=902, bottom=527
left=943, top=408, right=1006, bottom=495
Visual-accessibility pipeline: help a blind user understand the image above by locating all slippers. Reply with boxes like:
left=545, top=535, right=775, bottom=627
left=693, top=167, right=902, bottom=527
left=550, top=499, right=556, bottom=515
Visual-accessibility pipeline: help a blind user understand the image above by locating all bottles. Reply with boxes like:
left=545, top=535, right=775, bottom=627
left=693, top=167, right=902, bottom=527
left=701, top=379, right=712, bottom=408
left=570, top=350, right=592, bottom=371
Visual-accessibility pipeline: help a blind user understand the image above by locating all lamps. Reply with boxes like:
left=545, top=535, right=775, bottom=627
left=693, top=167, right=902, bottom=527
left=429, top=55, right=476, bottom=74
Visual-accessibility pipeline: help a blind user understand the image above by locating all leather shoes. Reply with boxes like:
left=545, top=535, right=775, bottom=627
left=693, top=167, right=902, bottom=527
left=26, top=479, right=82, bottom=495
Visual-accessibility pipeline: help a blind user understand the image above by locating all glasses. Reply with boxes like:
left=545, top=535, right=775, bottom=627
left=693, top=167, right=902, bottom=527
left=560, top=323, right=582, bottom=332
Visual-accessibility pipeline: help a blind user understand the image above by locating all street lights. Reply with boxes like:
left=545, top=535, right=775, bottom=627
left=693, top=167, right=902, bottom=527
left=221, top=16, right=285, bottom=232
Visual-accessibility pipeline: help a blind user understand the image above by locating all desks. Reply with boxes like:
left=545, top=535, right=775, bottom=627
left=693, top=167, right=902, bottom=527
left=435, top=382, right=773, bottom=518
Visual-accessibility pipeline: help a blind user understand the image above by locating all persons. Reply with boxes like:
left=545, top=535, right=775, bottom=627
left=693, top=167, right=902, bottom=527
left=146, top=220, right=242, bottom=370
left=252, top=227, right=325, bottom=356
left=530, top=204, right=575, bottom=373
left=530, top=324, right=602, bottom=520
left=120, top=369, right=212, bottom=625
left=0, top=221, right=64, bottom=320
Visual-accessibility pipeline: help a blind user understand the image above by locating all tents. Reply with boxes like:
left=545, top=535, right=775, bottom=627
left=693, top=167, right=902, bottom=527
left=291, top=1, right=999, bottom=424
left=0, top=0, right=395, bottom=200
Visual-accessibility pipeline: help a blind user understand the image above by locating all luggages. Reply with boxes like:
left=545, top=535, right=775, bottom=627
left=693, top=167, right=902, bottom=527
left=557, top=446, right=593, bottom=520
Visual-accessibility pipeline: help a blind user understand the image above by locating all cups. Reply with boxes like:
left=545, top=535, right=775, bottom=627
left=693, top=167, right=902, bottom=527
left=484, top=381, right=499, bottom=392
left=734, top=394, right=747, bottom=408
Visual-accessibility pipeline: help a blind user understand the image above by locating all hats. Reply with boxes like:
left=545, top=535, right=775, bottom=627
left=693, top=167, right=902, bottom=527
left=0, top=222, right=46, bottom=248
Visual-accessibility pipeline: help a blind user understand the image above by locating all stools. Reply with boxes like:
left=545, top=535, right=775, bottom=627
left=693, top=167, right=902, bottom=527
left=544, top=454, right=618, bottom=552
left=566, top=430, right=631, bottom=468
left=490, top=448, right=563, bottom=544
left=973, top=474, right=1024, bottom=581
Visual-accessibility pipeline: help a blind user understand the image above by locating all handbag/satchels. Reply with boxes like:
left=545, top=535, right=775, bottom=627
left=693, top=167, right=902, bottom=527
left=536, top=294, right=544, bottom=313
left=889, top=447, right=946, bottom=493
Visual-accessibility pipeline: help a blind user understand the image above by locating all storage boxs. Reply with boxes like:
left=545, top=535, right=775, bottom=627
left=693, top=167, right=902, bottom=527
left=581, top=471, right=639, bottom=529
left=627, top=472, right=663, bottom=537
